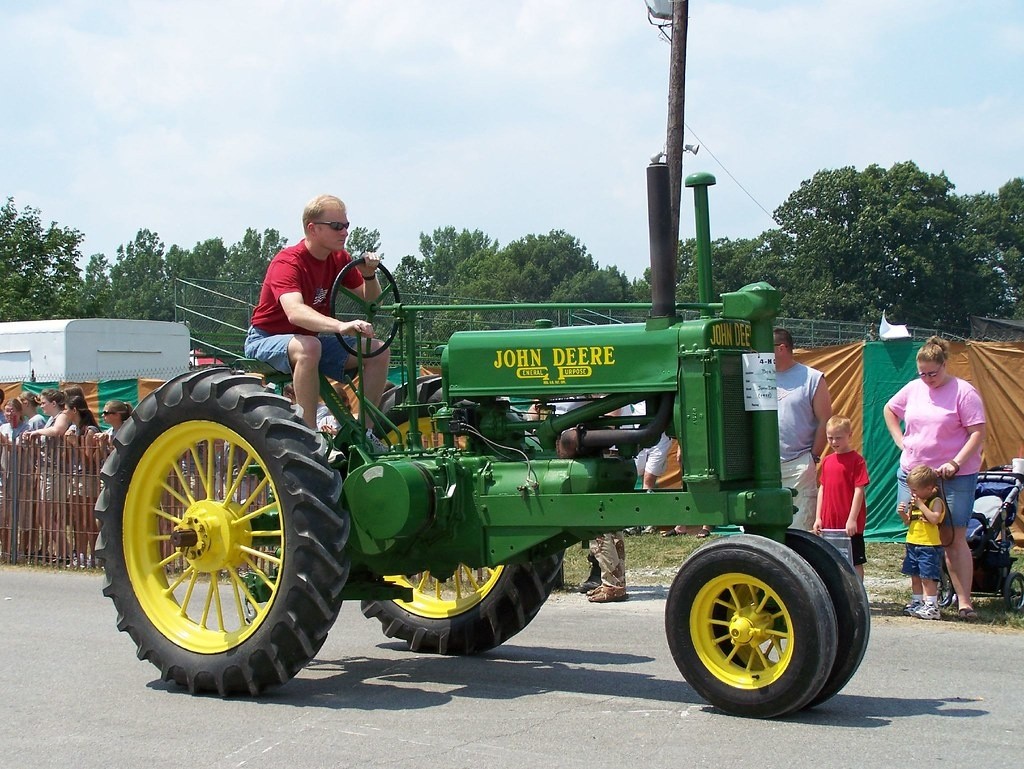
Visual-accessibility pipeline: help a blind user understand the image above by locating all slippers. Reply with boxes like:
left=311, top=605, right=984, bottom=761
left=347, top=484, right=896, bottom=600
left=697, top=529, right=710, bottom=538
left=661, top=529, right=687, bottom=538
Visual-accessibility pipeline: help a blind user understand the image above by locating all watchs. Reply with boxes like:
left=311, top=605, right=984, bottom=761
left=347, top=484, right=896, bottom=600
left=811, top=454, right=820, bottom=464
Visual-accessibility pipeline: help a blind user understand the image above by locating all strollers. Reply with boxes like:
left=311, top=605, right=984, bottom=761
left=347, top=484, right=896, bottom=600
left=936, top=465, right=1024, bottom=613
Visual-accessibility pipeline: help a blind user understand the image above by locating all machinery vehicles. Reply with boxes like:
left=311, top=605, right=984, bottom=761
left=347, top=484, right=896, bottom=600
left=94, top=163, right=872, bottom=721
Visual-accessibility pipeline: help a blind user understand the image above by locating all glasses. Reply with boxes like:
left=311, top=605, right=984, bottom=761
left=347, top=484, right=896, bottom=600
left=103, top=411, right=116, bottom=416
left=917, top=361, right=944, bottom=377
left=307, top=221, right=349, bottom=231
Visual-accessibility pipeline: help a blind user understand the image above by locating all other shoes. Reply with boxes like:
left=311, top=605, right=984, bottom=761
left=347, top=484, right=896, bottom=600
left=579, top=575, right=603, bottom=594
left=586, top=584, right=628, bottom=603
left=958, top=607, right=976, bottom=619
left=24, top=550, right=98, bottom=568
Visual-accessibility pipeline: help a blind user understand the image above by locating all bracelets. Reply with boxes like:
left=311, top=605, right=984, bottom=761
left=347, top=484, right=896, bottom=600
left=362, top=272, right=376, bottom=281
left=948, top=460, right=960, bottom=472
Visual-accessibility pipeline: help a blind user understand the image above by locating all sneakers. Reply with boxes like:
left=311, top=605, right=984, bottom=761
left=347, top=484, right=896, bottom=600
left=365, top=428, right=389, bottom=453
left=325, top=447, right=346, bottom=464
left=914, top=602, right=941, bottom=620
left=902, top=599, right=924, bottom=616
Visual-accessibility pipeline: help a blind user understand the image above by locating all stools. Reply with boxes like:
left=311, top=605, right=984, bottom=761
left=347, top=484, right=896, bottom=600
left=234, top=358, right=293, bottom=395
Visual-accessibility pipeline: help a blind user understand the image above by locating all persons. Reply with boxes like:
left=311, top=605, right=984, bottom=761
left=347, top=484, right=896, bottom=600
left=282, top=384, right=355, bottom=439
left=883, top=335, right=987, bottom=622
left=244, top=196, right=393, bottom=459
left=524, top=394, right=672, bottom=603
left=612, top=427, right=672, bottom=535
left=812, top=415, right=870, bottom=583
left=897, top=465, right=946, bottom=619
left=772, top=326, right=833, bottom=532
left=866, top=321, right=879, bottom=341
left=661, top=442, right=710, bottom=540
left=0, top=384, right=270, bottom=577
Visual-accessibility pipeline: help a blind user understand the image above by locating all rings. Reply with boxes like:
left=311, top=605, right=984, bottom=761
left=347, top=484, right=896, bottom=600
left=357, top=322, right=362, bottom=326
